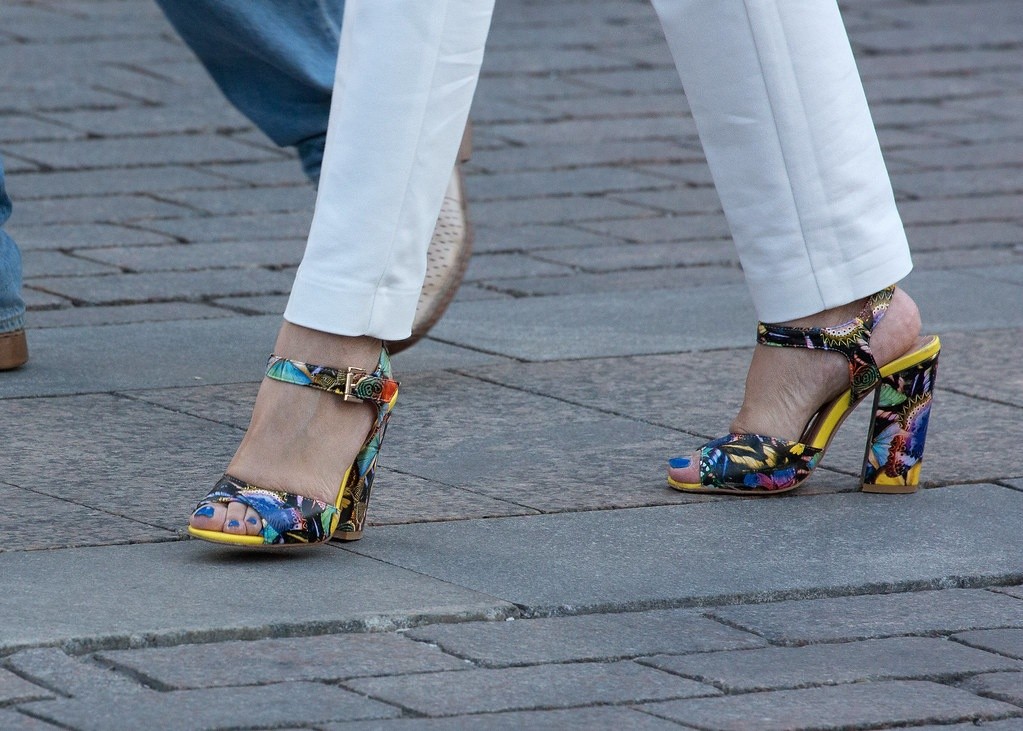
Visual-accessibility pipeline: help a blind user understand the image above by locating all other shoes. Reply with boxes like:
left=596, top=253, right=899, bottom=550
left=382, top=122, right=472, bottom=354
left=0, top=328, right=30, bottom=371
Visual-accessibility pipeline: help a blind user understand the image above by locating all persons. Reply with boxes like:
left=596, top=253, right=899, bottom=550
left=0, top=0, right=476, bottom=374
left=187, top=0, right=941, bottom=549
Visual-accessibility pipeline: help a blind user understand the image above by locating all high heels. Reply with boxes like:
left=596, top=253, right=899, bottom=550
left=668, top=285, right=941, bottom=495
left=188, top=348, right=401, bottom=551
